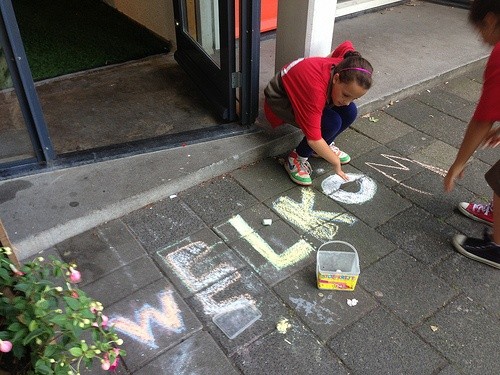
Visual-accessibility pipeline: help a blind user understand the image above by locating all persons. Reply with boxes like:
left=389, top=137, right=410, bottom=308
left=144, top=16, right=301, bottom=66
left=443, top=0, right=500, bottom=270
left=263, top=40, right=374, bottom=186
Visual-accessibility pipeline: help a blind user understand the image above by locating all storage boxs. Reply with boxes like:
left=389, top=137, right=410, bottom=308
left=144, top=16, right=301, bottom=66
left=316, top=249, right=360, bottom=291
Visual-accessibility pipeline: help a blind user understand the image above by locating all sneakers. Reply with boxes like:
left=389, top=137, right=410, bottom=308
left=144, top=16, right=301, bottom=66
left=451, top=227, right=500, bottom=270
left=284, top=148, right=313, bottom=185
left=457, top=200, right=495, bottom=227
left=310, top=142, right=351, bottom=164
